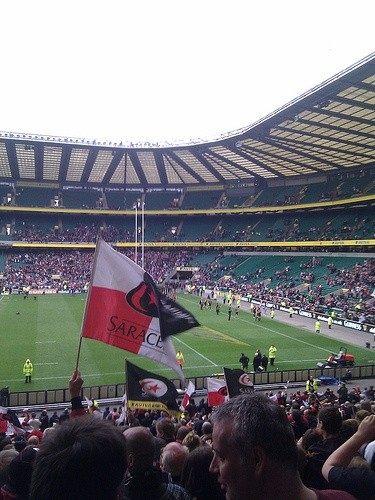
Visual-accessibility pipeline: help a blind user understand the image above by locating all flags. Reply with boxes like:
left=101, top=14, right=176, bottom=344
left=81, top=239, right=200, bottom=382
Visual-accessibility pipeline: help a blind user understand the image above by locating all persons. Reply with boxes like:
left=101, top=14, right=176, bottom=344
left=161, top=214, right=375, bottom=330
left=0, top=349, right=375, bottom=500
left=314, top=319, right=321, bottom=333
left=27, top=414, right=128, bottom=499
left=176, top=351, right=184, bottom=370
left=261, top=353, right=268, bottom=370
left=253, top=353, right=260, bottom=371
left=209, top=393, right=356, bottom=499
left=23, top=359, right=33, bottom=383
left=269, top=345, right=277, bottom=365
left=239, top=353, right=249, bottom=372
